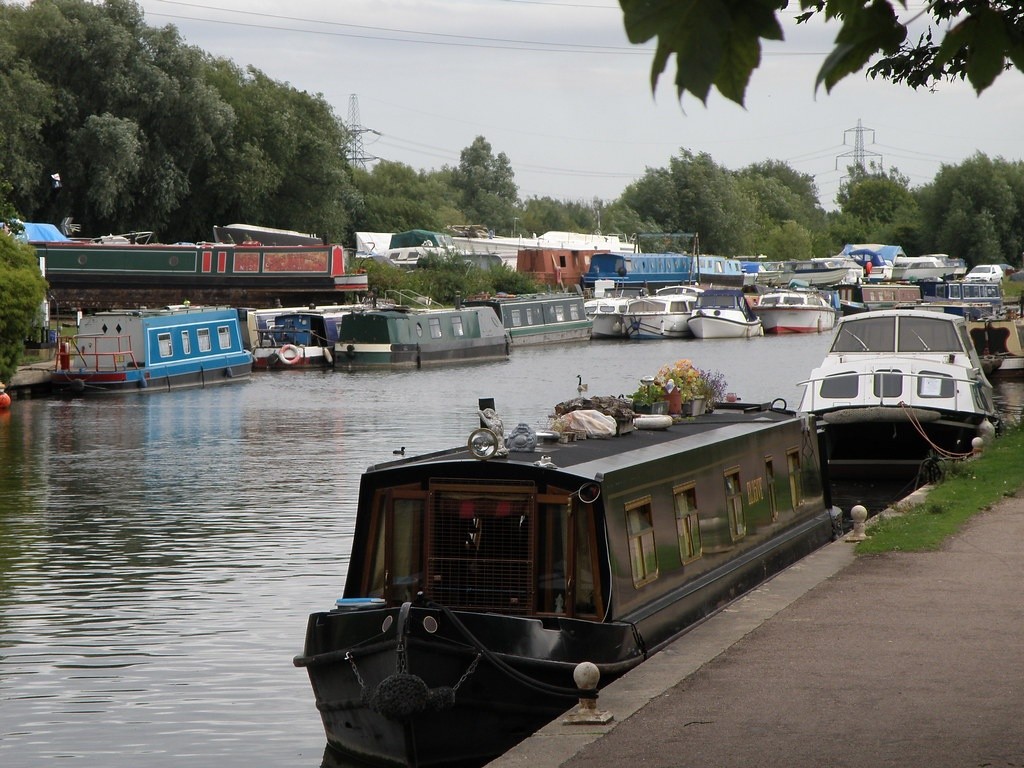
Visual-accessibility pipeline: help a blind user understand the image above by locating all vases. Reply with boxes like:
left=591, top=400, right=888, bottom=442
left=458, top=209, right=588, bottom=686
left=681, top=395, right=705, bottom=417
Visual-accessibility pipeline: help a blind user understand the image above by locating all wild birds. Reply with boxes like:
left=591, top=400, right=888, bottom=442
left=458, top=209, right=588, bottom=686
left=575, top=374, right=588, bottom=391
left=393, top=446, right=406, bottom=456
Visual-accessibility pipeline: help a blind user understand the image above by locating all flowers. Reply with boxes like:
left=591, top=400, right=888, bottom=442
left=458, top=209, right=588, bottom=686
left=654, top=358, right=704, bottom=404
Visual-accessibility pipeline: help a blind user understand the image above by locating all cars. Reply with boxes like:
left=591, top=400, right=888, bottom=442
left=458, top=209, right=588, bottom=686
left=999, top=263, right=1014, bottom=272
left=966, top=264, right=1004, bottom=284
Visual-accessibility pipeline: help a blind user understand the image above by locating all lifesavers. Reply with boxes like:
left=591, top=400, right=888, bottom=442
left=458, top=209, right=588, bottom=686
left=278, top=344, right=301, bottom=365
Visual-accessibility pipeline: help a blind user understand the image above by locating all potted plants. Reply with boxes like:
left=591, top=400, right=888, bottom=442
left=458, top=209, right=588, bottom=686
left=626, top=383, right=670, bottom=415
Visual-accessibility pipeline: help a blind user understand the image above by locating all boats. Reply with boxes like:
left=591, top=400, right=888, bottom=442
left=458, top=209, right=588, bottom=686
left=461, top=293, right=592, bottom=347
left=580, top=244, right=969, bottom=293
left=333, top=306, right=512, bottom=367
left=799, top=302, right=998, bottom=469
left=583, top=297, right=634, bottom=337
left=622, top=286, right=705, bottom=341
left=752, top=289, right=836, bottom=335
left=965, top=287, right=1023, bottom=376
left=236, top=303, right=296, bottom=349
left=51, top=306, right=259, bottom=394
left=212, top=222, right=323, bottom=246
left=386, top=225, right=642, bottom=272
left=248, top=311, right=351, bottom=371
left=288, top=396, right=840, bottom=768
left=686, top=289, right=763, bottom=339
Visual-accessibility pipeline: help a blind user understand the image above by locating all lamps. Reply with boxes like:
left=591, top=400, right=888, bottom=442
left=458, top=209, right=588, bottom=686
left=468, top=428, right=498, bottom=462
